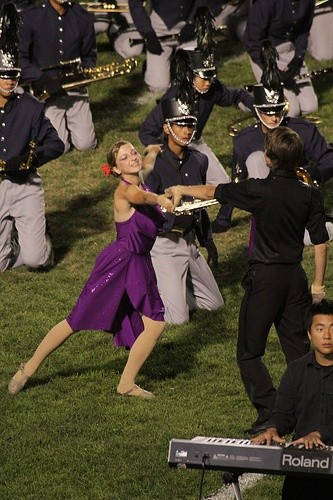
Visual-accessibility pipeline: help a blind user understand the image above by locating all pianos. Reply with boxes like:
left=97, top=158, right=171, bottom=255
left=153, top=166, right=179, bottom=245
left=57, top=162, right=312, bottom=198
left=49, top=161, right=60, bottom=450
left=167, top=435, right=333, bottom=500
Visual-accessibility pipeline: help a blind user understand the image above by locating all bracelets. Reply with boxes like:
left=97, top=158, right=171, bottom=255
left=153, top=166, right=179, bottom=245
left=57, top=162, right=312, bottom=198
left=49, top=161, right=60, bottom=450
left=310, top=284, right=326, bottom=295
left=265, top=426, right=279, bottom=434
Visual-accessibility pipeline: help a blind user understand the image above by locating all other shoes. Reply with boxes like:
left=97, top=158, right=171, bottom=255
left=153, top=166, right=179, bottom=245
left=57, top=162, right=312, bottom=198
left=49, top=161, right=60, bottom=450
left=117, top=384, right=157, bottom=400
left=309, top=283, right=326, bottom=295
left=243, top=424, right=267, bottom=438
left=8, top=362, right=32, bottom=395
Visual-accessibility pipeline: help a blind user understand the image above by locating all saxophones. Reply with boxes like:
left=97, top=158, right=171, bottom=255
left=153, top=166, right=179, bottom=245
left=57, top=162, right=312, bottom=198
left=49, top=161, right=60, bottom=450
left=0, top=139, right=37, bottom=171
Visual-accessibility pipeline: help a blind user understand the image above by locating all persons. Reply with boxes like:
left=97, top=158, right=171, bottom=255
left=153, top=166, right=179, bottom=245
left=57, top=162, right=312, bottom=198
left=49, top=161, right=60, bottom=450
left=230, top=85, right=333, bottom=243
left=100, top=0, right=243, bottom=97
left=9, top=140, right=174, bottom=400
left=248, top=300, right=333, bottom=500
left=18, top=0, right=100, bottom=154
left=143, top=102, right=223, bottom=327
left=139, top=56, right=244, bottom=184
left=0, top=52, right=65, bottom=271
left=242, top=0, right=319, bottom=117
left=164, top=126, right=328, bottom=438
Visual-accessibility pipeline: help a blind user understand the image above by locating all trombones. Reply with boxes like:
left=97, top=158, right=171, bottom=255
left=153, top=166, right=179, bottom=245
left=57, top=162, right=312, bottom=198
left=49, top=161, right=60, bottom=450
left=227, top=95, right=323, bottom=137
left=78, top=1, right=147, bottom=13
left=17, top=56, right=139, bottom=102
left=159, top=166, right=314, bottom=217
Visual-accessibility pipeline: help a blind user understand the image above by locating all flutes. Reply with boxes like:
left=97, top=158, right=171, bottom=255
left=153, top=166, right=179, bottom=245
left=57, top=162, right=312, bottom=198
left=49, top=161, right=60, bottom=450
left=243, top=67, right=333, bottom=91
left=129, top=27, right=221, bottom=48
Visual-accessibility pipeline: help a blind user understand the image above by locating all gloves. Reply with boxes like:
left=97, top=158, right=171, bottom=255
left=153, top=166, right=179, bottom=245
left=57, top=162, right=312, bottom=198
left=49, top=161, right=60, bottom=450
left=79, top=59, right=95, bottom=73
left=286, top=57, right=304, bottom=75
left=144, top=33, right=164, bottom=56
left=176, top=23, right=195, bottom=44
left=40, top=73, right=65, bottom=96
left=204, top=241, right=218, bottom=270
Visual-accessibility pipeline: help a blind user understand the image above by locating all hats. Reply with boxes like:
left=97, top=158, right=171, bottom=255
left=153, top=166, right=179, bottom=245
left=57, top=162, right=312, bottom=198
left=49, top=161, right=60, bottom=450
left=161, top=48, right=200, bottom=127
left=170, top=6, right=222, bottom=103
left=0, top=2, right=23, bottom=79
left=251, top=44, right=288, bottom=115
